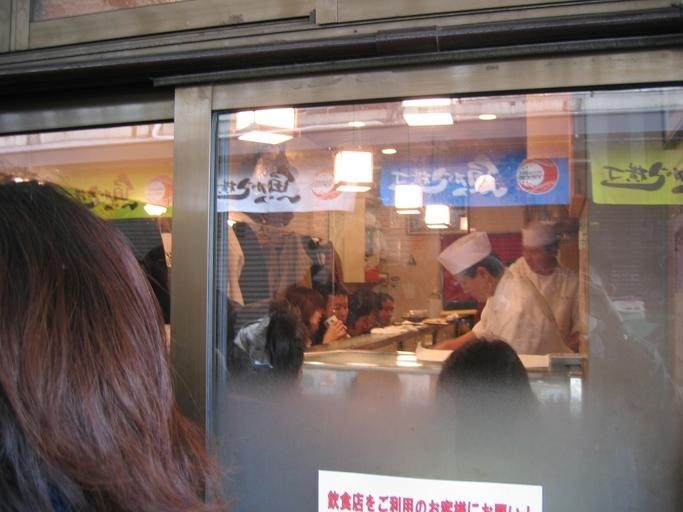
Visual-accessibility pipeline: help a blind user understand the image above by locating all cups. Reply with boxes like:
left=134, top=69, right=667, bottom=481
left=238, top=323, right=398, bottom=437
left=324, top=316, right=351, bottom=341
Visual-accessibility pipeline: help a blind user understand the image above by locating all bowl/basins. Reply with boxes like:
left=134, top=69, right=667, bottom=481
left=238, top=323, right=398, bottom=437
left=401, top=315, right=427, bottom=323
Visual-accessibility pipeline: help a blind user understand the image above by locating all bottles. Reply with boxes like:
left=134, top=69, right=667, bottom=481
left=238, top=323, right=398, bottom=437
left=429, top=288, right=441, bottom=320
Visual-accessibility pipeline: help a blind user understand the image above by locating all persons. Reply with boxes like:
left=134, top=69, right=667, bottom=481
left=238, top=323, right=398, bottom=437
left=584, top=221, right=682, bottom=512
left=417, top=336, right=573, bottom=481
left=428, top=231, right=577, bottom=355
left=226, top=213, right=394, bottom=407
left=502, top=220, right=581, bottom=355
left=0, top=173, right=235, bottom=512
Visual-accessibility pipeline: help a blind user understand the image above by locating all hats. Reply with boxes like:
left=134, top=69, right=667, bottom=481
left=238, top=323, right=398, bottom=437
left=521, top=220, right=558, bottom=248
left=438, top=231, right=491, bottom=274
